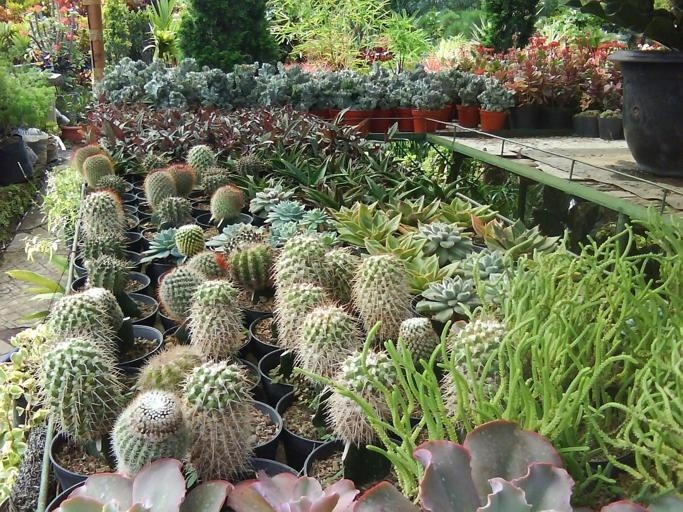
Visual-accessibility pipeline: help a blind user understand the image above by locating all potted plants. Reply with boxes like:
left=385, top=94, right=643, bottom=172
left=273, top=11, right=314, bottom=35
left=0, top=2, right=683, bottom=512
